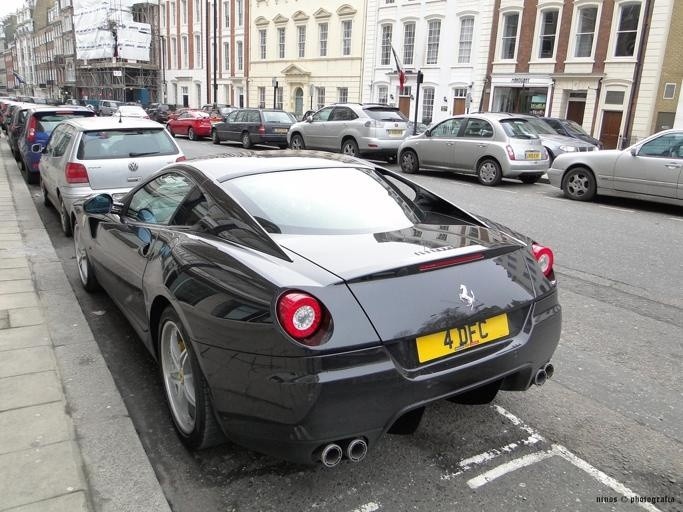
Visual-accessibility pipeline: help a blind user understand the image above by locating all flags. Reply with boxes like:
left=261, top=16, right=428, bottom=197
left=12, top=72, right=24, bottom=84
left=392, top=49, right=405, bottom=93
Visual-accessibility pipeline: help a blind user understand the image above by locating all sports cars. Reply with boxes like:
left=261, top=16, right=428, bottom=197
left=70, top=148, right=563, bottom=469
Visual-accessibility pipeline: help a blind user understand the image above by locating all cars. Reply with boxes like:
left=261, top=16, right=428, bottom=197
left=547, top=128, right=683, bottom=207
left=211, top=107, right=297, bottom=150
left=0, top=97, right=187, bottom=236
left=286, top=101, right=413, bottom=163
left=396, top=112, right=602, bottom=186
left=166, top=101, right=237, bottom=140
